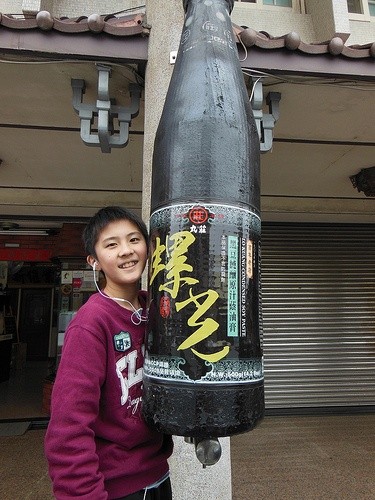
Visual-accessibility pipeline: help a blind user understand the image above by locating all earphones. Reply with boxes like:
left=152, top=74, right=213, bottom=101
left=93, top=262, right=98, bottom=270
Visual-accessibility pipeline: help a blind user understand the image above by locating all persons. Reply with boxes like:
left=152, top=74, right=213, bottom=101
left=44, top=206, right=174, bottom=500
